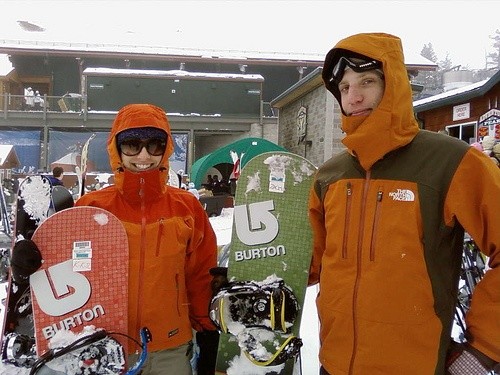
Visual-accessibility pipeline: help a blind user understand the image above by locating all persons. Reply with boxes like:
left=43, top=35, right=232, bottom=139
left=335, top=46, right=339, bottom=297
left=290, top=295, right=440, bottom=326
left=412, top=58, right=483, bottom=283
left=10, top=104, right=219, bottom=375
left=208, top=32, right=500, bottom=375
left=205, top=174, right=220, bottom=188
left=2, top=167, right=102, bottom=199
left=25, top=85, right=73, bottom=106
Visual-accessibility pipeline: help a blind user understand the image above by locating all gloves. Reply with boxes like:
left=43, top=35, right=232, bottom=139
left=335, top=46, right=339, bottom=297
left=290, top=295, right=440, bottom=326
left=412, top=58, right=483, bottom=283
left=209, top=267, right=229, bottom=297
left=444, top=342, right=500, bottom=375
left=195, top=329, right=220, bottom=375
left=12, top=239, right=42, bottom=275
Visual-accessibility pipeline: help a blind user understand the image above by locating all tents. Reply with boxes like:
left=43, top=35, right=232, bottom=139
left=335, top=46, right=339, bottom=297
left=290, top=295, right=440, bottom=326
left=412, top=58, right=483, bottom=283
left=190, top=137, right=290, bottom=190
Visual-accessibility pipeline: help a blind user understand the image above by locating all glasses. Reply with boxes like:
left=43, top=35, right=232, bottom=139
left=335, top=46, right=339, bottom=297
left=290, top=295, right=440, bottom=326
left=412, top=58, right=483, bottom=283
left=119, top=135, right=166, bottom=157
left=329, top=51, right=381, bottom=87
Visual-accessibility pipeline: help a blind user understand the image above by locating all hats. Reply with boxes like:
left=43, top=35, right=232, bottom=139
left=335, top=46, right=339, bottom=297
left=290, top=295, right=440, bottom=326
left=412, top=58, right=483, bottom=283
left=116, top=127, right=168, bottom=139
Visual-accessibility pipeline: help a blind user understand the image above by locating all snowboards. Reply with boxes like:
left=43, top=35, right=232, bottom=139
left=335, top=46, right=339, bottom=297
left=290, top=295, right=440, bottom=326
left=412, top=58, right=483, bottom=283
left=30, top=206, right=130, bottom=375
left=215, top=151, right=319, bottom=375
left=0, top=174, right=53, bottom=368
left=52, top=186, right=74, bottom=212
